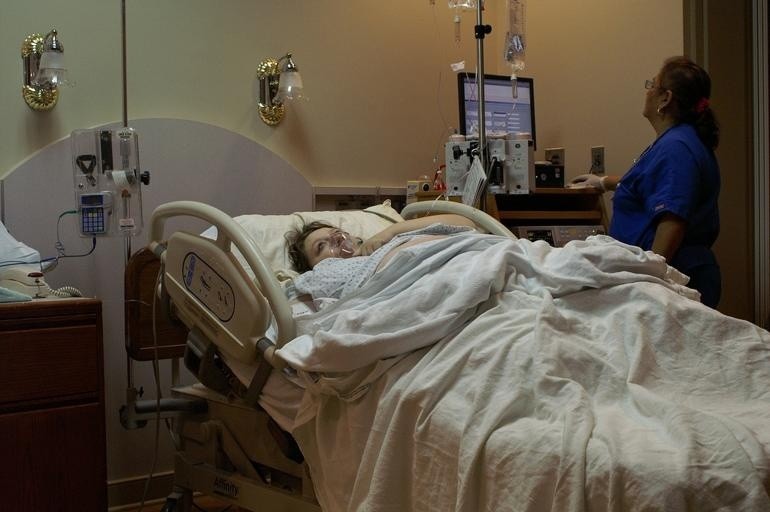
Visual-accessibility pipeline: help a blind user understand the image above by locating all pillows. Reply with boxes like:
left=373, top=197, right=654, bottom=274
left=196, top=197, right=407, bottom=349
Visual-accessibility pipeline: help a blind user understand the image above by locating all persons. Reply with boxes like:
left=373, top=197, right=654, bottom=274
left=286, top=213, right=476, bottom=306
left=569, top=54, right=722, bottom=313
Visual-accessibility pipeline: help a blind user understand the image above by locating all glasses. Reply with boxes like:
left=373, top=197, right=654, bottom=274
left=645, top=79, right=655, bottom=88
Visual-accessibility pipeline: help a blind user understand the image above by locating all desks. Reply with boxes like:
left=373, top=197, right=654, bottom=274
left=411, top=185, right=622, bottom=255
left=1, top=296, right=113, bottom=511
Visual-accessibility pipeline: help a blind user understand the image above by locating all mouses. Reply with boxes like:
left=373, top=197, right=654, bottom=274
left=567, top=180, right=587, bottom=188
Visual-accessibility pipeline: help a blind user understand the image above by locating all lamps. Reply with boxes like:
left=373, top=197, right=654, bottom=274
left=16, top=27, right=79, bottom=114
left=251, top=48, right=320, bottom=130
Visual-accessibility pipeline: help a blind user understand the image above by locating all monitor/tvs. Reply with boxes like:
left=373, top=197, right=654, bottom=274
left=458, top=72, right=536, bottom=151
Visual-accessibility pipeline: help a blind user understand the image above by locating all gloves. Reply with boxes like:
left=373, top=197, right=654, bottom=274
left=571, top=174, right=607, bottom=190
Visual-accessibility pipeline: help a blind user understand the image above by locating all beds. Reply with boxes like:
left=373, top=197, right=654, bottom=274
left=129, top=192, right=770, bottom=511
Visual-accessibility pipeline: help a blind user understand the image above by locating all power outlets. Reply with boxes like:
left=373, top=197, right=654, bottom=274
left=545, top=146, right=565, bottom=168
left=591, top=145, right=606, bottom=174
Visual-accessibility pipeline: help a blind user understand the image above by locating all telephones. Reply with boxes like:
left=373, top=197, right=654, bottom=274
left=0, top=266, right=84, bottom=298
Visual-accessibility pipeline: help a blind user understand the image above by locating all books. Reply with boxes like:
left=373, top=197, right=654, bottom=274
left=459, top=153, right=488, bottom=210
